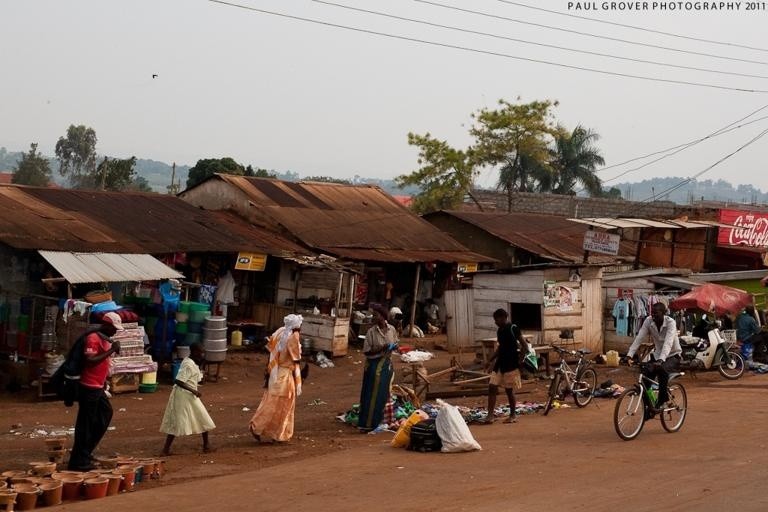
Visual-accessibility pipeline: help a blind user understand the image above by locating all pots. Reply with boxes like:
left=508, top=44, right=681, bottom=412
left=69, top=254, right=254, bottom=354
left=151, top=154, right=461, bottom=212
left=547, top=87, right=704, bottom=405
left=300, top=336, right=314, bottom=349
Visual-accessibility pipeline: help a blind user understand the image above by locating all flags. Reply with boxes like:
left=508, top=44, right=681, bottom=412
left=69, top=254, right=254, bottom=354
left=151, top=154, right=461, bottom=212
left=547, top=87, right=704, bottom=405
left=0, top=437, right=162, bottom=512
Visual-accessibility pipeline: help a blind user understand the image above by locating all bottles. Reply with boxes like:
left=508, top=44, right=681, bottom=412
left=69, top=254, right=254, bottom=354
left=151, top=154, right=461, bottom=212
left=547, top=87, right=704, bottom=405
left=102, top=311, right=124, bottom=331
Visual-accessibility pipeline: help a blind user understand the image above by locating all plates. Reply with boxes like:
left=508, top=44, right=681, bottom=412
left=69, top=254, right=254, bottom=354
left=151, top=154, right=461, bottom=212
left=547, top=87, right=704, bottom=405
left=291, top=267, right=356, bottom=358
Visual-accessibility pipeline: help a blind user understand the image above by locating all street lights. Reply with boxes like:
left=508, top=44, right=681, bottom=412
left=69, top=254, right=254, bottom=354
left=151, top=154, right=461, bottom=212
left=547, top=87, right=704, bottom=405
left=522, top=351, right=540, bottom=372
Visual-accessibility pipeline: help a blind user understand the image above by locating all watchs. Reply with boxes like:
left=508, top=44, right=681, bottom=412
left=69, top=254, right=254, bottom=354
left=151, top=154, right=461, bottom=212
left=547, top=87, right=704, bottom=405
left=680, top=307, right=745, bottom=380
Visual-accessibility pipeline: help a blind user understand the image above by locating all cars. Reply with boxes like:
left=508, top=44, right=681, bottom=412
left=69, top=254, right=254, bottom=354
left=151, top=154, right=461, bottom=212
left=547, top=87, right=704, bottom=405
left=1, top=458, right=165, bottom=511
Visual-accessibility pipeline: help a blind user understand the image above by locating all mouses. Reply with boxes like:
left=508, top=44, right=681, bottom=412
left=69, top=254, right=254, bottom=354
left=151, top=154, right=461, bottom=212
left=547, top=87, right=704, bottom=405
left=617, top=290, right=682, bottom=302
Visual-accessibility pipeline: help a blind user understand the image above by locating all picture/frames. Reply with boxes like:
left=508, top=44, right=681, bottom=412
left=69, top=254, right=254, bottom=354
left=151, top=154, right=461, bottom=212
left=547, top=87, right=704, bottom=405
left=479, top=332, right=536, bottom=375
left=530, top=343, right=555, bottom=376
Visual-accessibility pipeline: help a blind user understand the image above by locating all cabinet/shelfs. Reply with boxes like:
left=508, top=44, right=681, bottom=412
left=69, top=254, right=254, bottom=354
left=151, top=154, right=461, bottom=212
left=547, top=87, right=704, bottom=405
left=230, top=328, right=243, bottom=346
left=647, top=388, right=658, bottom=403
left=331, top=307, right=336, bottom=317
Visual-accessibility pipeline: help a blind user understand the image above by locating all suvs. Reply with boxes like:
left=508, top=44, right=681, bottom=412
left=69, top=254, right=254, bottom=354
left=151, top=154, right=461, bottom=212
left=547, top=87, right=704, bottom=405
left=142, top=371, right=157, bottom=385
left=171, top=361, right=182, bottom=383
left=176, top=346, right=191, bottom=359
left=172, top=300, right=211, bottom=345
left=231, top=330, right=243, bottom=347
left=606, top=348, right=620, bottom=369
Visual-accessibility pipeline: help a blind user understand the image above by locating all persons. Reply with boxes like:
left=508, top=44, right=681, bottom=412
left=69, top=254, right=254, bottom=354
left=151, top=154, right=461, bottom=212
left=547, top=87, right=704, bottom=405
left=482, top=308, right=528, bottom=424
left=248, top=313, right=305, bottom=446
left=692, top=305, right=764, bottom=342
left=356, top=304, right=401, bottom=433
left=624, top=302, right=685, bottom=421
left=159, top=342, right=217, bottom=458
left=390, top=284, right=440, bottom=336
left=68, top=313, right=124, bottom=471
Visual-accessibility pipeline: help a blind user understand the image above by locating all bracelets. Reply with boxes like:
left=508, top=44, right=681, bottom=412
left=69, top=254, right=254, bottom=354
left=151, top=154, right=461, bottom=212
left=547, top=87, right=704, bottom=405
left=140, top=382, right=159, bottom=392
left=84, top=290, right=113, bottom=304
left=339, top=309, right=347, bottom=316
left=176, top=300, right=227, bottom=361
left=45, top=438, right=66, bottom=452
left=135, top=289, right=151, bottom=297
left=242, top=340, right=253, bottom=346
left=45, top=451, right=70, bottom=468
